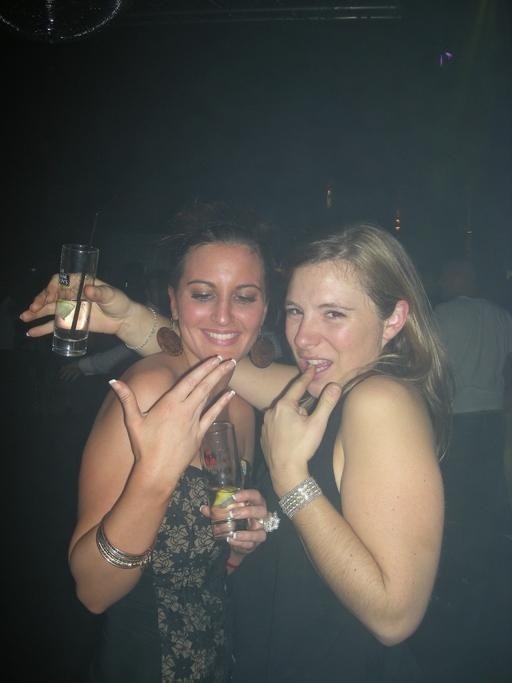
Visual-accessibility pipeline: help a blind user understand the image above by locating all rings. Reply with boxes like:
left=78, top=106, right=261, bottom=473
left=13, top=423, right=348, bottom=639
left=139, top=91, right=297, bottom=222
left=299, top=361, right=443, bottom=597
left=257, top=504, right=279, bottom=532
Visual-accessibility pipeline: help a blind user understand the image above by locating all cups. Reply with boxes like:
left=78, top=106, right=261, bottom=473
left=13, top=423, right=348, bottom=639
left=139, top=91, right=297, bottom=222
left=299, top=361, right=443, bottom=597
left=196, top=419, right=250, bottom=539
left=52, top=242, right=99, bottom=358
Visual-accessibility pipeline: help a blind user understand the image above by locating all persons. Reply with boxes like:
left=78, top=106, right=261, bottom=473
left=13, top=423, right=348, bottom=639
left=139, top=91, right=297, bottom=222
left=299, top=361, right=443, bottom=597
left=424, top=250, right=512, bottom=525
left=64, top=225, right=309, bottom=682
left=18, top=222, right=447, bottom=682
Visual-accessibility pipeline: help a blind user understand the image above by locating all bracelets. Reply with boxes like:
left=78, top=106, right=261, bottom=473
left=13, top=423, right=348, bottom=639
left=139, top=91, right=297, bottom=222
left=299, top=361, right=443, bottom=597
left=279, top=477, right=323, bottom=520
left=95, top=518, right=154, bottom=569
left=124, top=303, right=159, bottom=351
